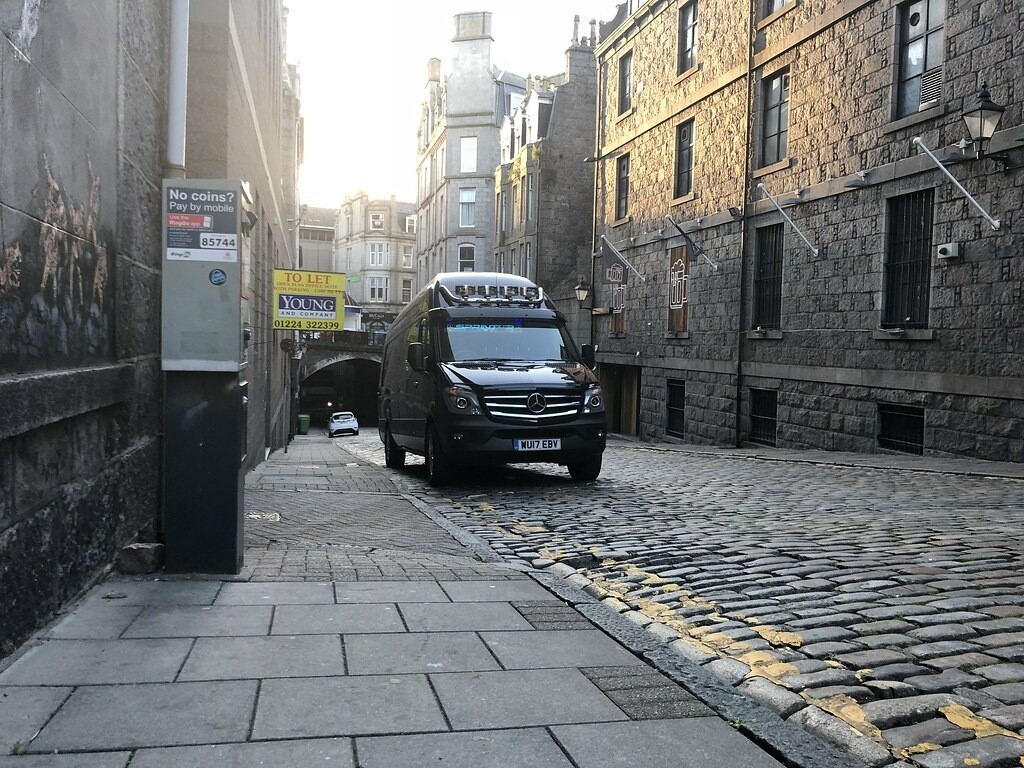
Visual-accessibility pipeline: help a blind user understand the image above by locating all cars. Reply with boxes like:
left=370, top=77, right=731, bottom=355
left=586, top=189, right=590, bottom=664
left=328, top=411, right=359, bottom=438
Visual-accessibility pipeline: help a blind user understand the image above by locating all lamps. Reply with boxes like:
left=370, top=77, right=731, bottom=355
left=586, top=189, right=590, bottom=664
left=572, top=276, right=613, bottom=316
left=582, top=157, right=597, bottom=163
left=959, top=80, right=1010, bottom=177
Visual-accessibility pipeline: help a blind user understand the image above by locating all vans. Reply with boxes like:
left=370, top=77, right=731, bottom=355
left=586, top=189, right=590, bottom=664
left=379, top=270, right=609, bottom=489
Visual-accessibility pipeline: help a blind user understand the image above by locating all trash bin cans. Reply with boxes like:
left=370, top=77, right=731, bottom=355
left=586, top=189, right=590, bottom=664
left=298, top=414, right=310, bottom=435
